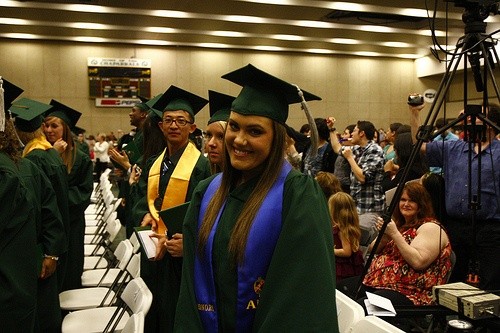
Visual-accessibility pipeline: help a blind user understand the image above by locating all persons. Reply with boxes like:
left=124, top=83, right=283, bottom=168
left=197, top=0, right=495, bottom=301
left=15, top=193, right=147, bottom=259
left=275, top=117, right=500, bottom=286
left=172, top=63, right=340, bottom=332
left=354, top=180, right=454, bottom=332
left=0, top=76, right=66, bottom=333
left=2, top=85, right=236, bottom=332
left=409, top=93, right=500, bottom=280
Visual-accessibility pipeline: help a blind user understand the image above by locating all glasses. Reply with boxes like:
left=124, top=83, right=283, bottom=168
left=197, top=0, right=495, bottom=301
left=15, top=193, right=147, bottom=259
left=162, top=115, right=192, bottom=126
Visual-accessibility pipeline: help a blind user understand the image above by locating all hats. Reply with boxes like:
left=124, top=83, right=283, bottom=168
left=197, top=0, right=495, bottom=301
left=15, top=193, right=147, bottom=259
left=0, top=76, right=24, bottom=132
left=208, top=89, right=236, bottom=124
left=135, top=94, right=149, bottom=111
left=151, top=85, right=208, bottom=123
left=221, top=64, right=322, bottom=158
left=71, top=126, right=85, bottom=136
left=145, top=93, right=163, bottom=124
left=47, top=99, right=82, bottom=131
left=8, top=97, right=53, bottom=147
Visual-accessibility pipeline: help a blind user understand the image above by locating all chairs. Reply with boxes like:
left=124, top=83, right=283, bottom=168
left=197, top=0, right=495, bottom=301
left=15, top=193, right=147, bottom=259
left=55, top=168, right=458, bottom=333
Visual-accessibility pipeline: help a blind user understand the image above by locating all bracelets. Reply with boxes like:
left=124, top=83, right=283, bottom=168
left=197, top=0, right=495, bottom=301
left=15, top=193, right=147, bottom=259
left=328, top=126, right=335, bottom=132
left=42, top=254, right=60, bottom=260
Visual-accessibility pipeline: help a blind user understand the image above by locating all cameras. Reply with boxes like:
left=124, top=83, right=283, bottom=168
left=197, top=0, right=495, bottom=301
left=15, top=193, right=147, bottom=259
left=408, top=95, right=423, bottom=106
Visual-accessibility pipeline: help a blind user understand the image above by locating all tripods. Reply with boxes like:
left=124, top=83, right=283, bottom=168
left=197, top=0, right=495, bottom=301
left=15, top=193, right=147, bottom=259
left=351, top=22, right=500, bottom=300
